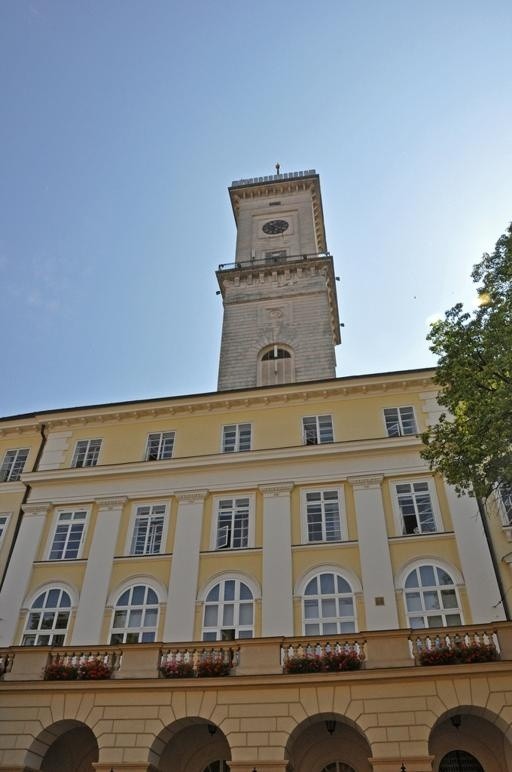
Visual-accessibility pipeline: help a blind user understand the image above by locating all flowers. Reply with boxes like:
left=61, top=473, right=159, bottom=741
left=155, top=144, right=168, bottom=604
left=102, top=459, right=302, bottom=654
left=283, top=653, right=362, bottom=674
left=419, top=640, right=502, bottom=665
left=39, top=655, right=116, bottom=681
left=160, top=657, right=230, bottom=678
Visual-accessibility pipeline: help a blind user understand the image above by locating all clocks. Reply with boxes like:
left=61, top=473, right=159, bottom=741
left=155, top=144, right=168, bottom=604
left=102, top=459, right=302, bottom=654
left=258, top=217, right=294, bottom=237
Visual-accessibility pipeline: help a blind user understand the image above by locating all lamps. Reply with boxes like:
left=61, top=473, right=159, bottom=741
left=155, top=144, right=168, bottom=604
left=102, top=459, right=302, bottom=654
left=453, top=715, right=465, bottom=732
left=325, top=721, right=338, bottom=735
left=207, top=725, right=219, bottom=737
